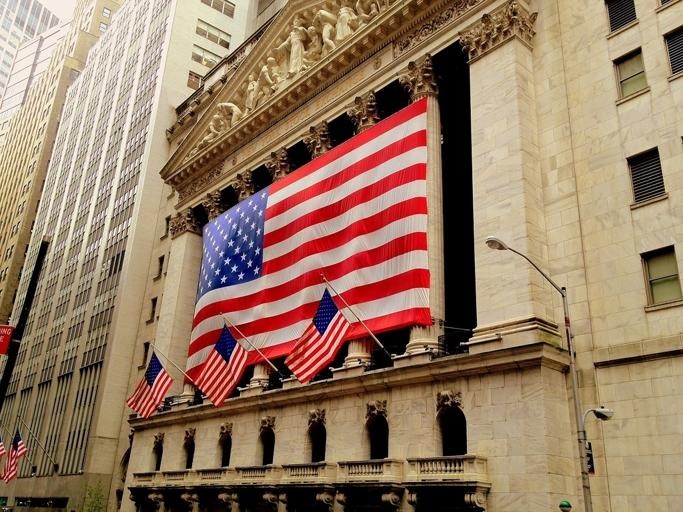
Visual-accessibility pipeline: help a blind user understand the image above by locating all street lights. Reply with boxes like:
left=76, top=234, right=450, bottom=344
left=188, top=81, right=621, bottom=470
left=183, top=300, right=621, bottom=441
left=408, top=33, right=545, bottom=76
left=482, top=234, right=616, bottom=512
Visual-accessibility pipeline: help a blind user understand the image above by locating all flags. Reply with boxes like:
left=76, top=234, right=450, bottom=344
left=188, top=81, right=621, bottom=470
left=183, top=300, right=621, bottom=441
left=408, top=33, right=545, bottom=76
left=281, top=286, right=354, bottom=386
left=123, top=350, right=174, bottom=421
left=183, top=90, right=432, bottom=382
left=189, top=323, right=250, bottom=411
left=0, top=432, right=6, bottom=459
left=0, top=426, right=28, bottom=484
